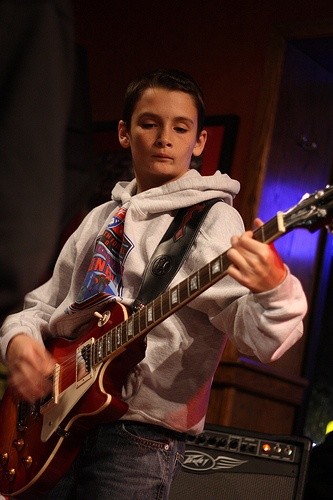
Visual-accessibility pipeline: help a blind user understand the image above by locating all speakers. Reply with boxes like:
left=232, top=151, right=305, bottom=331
left=174, top=422, right=312, bottom=500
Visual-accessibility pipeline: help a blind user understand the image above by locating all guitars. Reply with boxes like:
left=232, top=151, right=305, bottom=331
left=0, top=177, right=332, bottom=497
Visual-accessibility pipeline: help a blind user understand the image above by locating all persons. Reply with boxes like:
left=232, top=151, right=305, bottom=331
left=1, top=71, right=308, bottom=499
